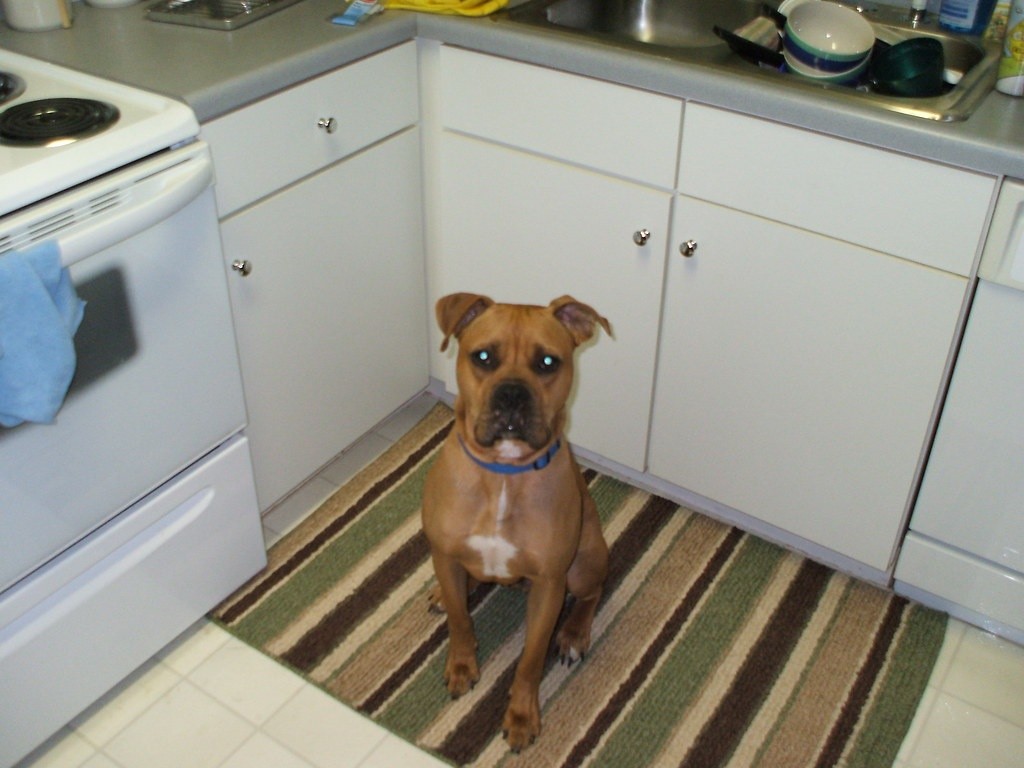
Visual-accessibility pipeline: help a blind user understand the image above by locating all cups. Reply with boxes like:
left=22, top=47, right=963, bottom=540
left=1, top=0, right=75, bottom=30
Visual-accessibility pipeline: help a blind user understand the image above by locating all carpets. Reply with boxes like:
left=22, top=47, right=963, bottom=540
left=204, top=400, right=950, bottom=768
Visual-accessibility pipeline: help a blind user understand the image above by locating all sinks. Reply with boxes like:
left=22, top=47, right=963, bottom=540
left=744, top=12, right=986, bottom=100
left=512, top=0, right=767, bottom=62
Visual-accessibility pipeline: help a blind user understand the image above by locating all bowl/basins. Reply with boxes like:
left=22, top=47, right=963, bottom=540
left=783, top=1, right=945, bottom=97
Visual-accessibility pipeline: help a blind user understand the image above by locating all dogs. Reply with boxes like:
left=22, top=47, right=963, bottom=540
left=419, top=292, right=615, bottom=754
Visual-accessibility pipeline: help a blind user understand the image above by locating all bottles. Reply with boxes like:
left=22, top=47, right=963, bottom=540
left=939, top=0, right=997, bottom=34
left=995, top=0, right=1024, bottom=97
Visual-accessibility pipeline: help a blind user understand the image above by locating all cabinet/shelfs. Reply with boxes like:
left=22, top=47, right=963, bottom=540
left=197, top=37, right=1024, bottom=648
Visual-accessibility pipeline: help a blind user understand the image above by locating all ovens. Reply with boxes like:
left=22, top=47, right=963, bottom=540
left=1, top=138, right=246, bottom=587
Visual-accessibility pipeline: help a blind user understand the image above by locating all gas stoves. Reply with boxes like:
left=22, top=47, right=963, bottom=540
left=1, top=40, right=199, bottom=214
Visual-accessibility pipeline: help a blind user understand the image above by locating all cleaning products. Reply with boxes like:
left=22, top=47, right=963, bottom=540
left=908, top=0, right=1024, bottom=98
